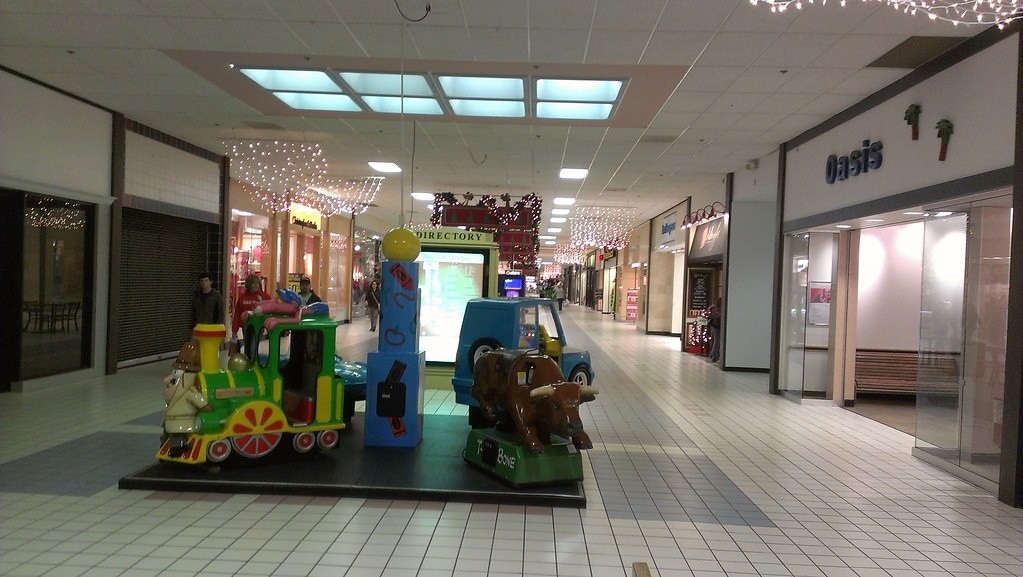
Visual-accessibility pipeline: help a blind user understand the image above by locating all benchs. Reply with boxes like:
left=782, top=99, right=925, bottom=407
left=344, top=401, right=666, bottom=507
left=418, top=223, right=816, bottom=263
left=855, top=349, right=961, bottom=405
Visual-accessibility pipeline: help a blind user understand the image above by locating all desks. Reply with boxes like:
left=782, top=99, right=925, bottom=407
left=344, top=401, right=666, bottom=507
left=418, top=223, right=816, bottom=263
left=30, top=303, right=63, bottom=333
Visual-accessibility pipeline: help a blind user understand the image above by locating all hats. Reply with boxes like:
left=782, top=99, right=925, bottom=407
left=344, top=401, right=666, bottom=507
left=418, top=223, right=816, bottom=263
left=300, top=276, right=310, bottom=284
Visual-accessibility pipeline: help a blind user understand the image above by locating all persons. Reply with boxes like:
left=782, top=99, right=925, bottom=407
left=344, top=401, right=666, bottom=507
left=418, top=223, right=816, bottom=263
left=706, top=297, right=721, bottom=362
left=232, top=274, right=271, bottom=367
left=554, top=284, right=565, bottom=314
left=189, top=272, right=225, bottom=336
left=364, top=281, right=382, bottom=332
left=296, top=276, right=323, bottom=307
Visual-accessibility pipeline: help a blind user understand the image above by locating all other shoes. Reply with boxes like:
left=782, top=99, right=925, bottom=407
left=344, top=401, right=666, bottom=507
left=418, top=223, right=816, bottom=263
left=369, top=328, right=376, bottom=332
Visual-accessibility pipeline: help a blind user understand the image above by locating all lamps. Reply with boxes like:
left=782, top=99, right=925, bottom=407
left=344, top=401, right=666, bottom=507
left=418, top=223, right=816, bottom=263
left=699, top=204, right=723, bottom=222
left=680, top=215, right=695, bottom=229
left=381, top=11, right=421, bottom=261
left=708, top=201, right=726, bottom=220
left=692, top=209, right=711, bottom=225
left=686, top=212, right=702, bottom=228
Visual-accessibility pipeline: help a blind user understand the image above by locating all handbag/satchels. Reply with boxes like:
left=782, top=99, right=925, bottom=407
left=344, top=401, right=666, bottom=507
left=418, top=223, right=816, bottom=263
left=378, top=303, right=380, bottom=311
left=228, top=334, right=241, bottom=357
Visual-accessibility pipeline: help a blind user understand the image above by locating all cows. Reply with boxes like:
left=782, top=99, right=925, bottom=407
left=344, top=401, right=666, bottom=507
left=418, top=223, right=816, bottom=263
left=470, top=348, right=600, bottom=449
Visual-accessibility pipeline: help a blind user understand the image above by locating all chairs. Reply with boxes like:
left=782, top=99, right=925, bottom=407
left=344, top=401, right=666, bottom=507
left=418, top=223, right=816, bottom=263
left=24, top=300, right=80, bottom=334
